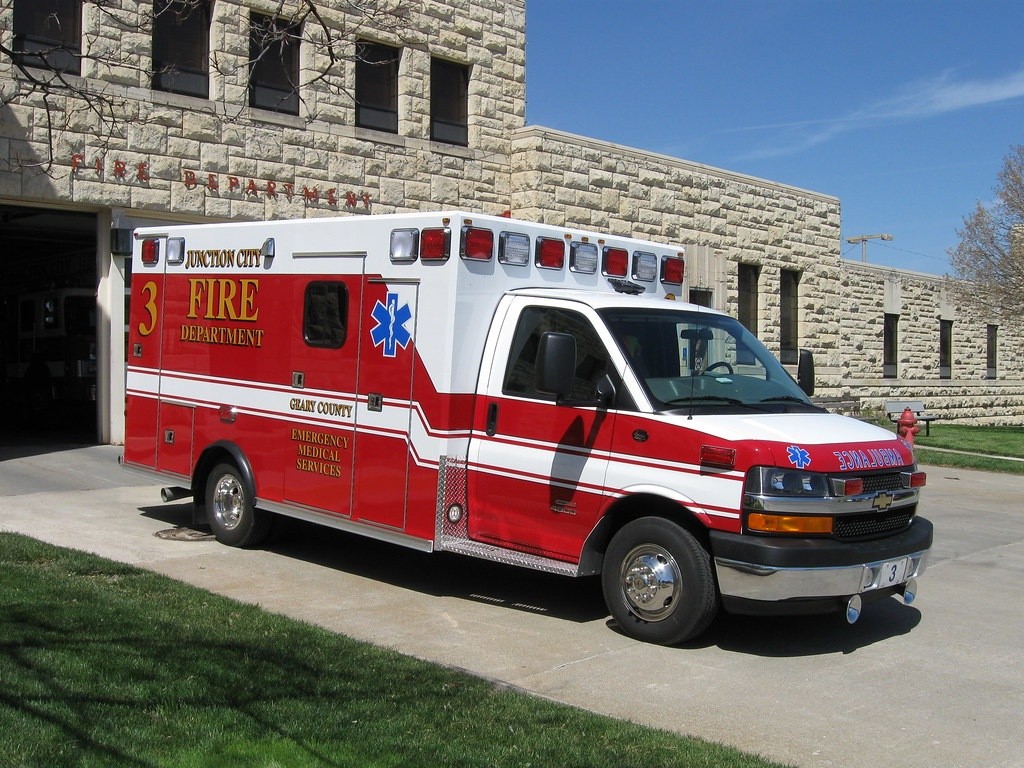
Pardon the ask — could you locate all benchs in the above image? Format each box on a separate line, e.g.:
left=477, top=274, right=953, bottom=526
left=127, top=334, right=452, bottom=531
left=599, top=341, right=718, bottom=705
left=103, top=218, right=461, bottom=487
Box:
left=886, top=400, right=939, bottom=436
left=809, top=396, right=878, bottom=422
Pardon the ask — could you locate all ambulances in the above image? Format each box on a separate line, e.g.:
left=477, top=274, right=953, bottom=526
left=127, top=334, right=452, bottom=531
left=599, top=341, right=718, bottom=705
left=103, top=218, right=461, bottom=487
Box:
left=122, top=212, right=935, bottom=648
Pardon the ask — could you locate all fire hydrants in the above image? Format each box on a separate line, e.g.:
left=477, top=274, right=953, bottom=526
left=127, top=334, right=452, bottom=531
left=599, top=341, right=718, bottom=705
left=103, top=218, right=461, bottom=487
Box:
left=895, top=406, right=922, bottom=456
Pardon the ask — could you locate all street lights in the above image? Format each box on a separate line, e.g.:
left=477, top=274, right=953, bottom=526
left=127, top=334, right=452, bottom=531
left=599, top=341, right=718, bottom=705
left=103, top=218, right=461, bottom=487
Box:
left=843, top=233, right=894, bottom=264
